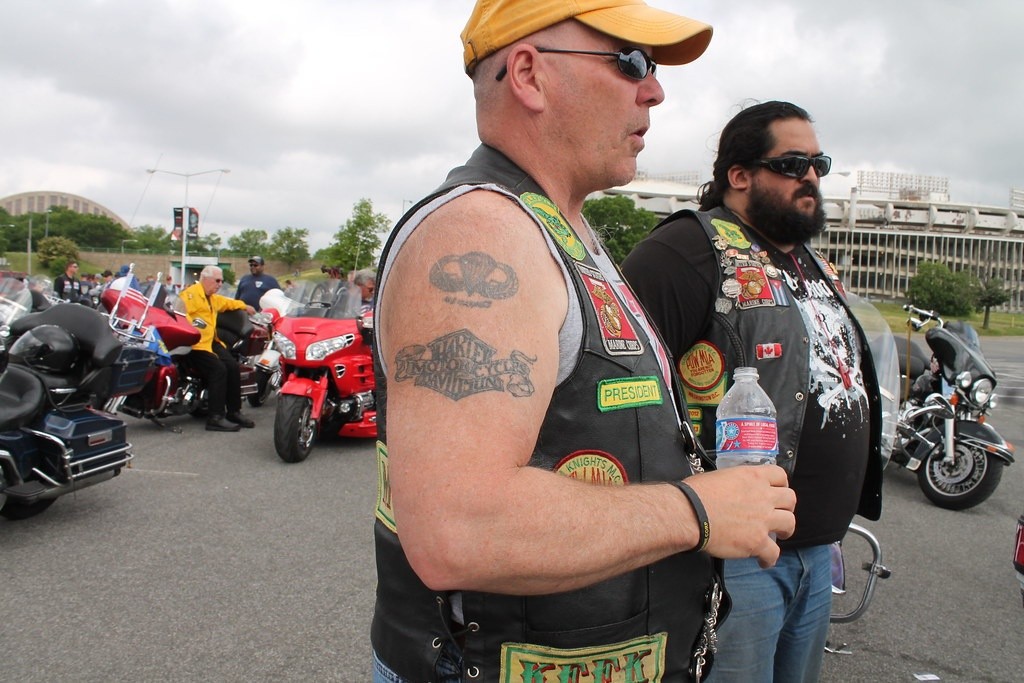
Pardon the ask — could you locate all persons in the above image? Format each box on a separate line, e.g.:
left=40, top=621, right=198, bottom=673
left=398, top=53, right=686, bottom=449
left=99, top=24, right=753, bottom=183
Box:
left=622, top=101, right=882, bottom=683
left=365, top=0, right=798, bottom=683
left=162, top=274, right=180, bottom=296
left=80, top=271, right=102, bottom=307
left=235, top=256, right=281, bottom=313
left=146, top=272, right=155, bottom=282
left=120, top=266, right=142, bottom=291
left=177, top=265, right=255, bottom=431
left=101, top=270, right=120, bottom=291
left=346, top=269, right=375, bottom=329
left=54, top=261, right=82, bottom=303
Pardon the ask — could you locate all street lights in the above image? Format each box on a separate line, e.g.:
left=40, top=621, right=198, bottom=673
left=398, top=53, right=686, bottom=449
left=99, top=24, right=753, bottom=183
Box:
left=146, top=168, right=231, bottom=293
left=402, top=199, right=413, bottom=216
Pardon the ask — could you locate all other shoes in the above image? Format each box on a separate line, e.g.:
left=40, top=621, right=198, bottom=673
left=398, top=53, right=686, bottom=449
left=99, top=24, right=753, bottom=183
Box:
left=226, top=411, right=254, bottom=428
left=206, top=417, right=240, bottom=431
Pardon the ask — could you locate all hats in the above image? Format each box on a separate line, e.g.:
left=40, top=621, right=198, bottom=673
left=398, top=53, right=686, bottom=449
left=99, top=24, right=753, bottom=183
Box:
left=120, top=265, right=129, bottom=276
left=249, top=255, right=265, bottom=265
left=461, top=0, right=714, bottom=81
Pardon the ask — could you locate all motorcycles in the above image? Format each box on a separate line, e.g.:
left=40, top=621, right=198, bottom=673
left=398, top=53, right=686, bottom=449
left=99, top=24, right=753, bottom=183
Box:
left=0, top=301, right=155, bottom=521
left=97, top=262, right=283, bottom=434
left=814, top=288, right=900, bottom=652
left=868, top=302, right=1016, bottom=511
left=260, top=272, right=379, bottom=463
left=0, top=265, right=331, bottom=375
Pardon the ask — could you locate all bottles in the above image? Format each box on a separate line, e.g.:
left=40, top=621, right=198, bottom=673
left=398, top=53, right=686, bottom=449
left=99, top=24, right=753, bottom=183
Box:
left=715, top=367, right=779, bottom=546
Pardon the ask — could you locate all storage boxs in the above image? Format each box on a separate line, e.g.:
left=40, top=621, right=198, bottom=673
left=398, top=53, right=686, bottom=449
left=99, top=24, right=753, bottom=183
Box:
left=42, top=409, right=126, bottom=474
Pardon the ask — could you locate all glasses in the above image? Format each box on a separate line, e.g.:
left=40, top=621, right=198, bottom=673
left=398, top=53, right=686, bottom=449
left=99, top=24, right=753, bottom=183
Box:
left=250, top=264, right=260, bottom=267
left=496, top=47, right=658, bottom=84
left=207, top=277, right=224, bottom=284
left=757, top=154, right=831, bottom=179
left=362, top=285, right=374, bottom=292
left=70, top=266, right=77, bottom=269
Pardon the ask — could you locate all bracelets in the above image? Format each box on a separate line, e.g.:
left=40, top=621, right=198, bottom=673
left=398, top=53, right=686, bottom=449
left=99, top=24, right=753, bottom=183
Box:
left=669, top=479, right=710, bottom=553
